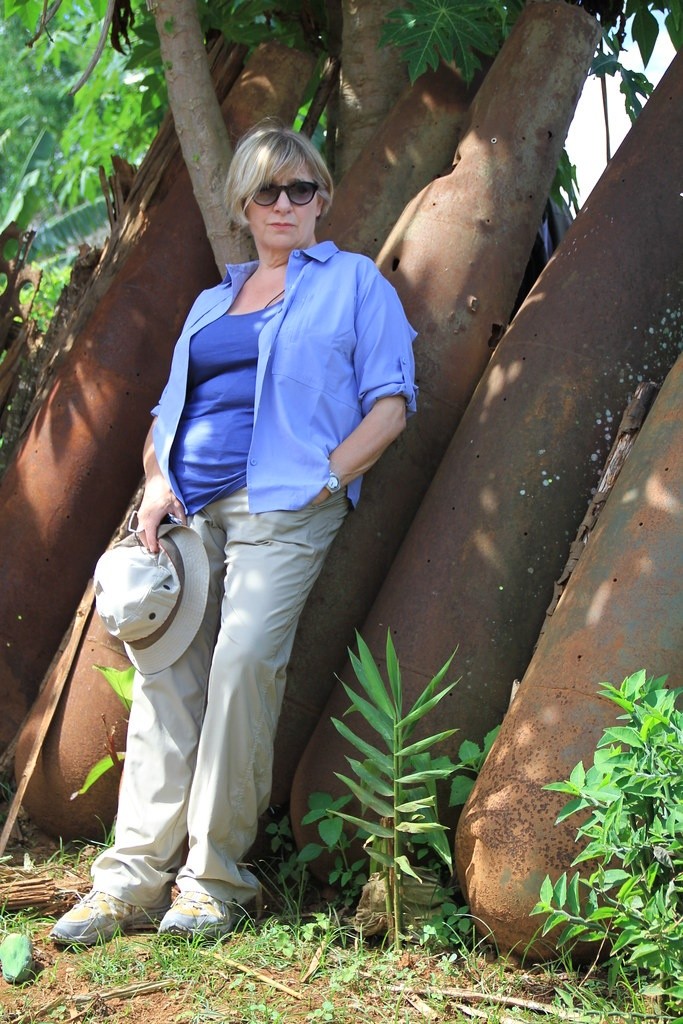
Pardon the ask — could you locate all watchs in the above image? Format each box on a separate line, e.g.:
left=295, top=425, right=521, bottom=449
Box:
left=324, top=470, right=341, bottom=496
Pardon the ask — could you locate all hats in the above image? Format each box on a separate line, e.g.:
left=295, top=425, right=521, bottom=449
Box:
left=93, top=524, right=210, bottom=675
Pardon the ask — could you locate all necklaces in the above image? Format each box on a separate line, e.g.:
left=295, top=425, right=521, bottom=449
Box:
left=264, top=290, right=285, bottom=308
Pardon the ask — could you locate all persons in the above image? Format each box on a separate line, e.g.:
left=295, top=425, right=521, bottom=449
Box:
left=49, top=116, right=418, bottom=946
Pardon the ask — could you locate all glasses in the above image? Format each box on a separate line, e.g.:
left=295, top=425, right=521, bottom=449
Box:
left=252, top=179, right=319, bottom=207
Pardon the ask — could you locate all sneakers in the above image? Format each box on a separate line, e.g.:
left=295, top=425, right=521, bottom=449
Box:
left=48, top=888, right=172, bottom=945
left=156, top=890, right=236, bottom=938
